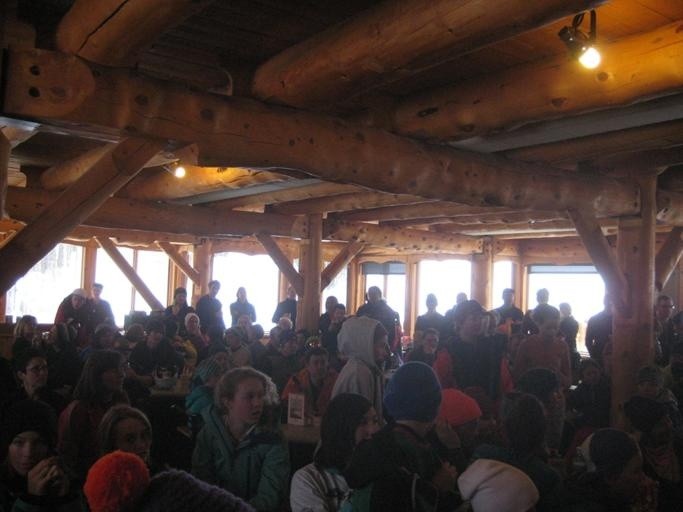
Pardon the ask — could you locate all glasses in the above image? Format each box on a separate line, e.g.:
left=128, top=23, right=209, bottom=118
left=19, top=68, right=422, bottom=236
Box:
left=31, top=365, right=48, bottom=373
left=661, top=305, right=676, bottom=309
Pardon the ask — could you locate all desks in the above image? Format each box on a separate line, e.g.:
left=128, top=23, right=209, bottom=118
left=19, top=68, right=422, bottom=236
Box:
left=146, top=374, right=192, bottom=401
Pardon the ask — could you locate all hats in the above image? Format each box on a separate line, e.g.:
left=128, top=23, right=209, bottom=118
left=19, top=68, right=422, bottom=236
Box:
left=72, top=288, right=87, bottom=299
left=517, top=369, right=561, bottom=408
left=173, top=287, right=187, bottom=299
left=199, top=358, right=222, bottom=384
left=454, top=299, right=491, bottom=324
left=383, top=361, right=442, bottom=423
left=623, top=396, right=669, bottom=432
left=225, top=325, right=245, bottom=339
left=434, top=388, right=483, bottom=427
left=0, top=398, right=57, bottom=463
left=636, top=365, right=665, bottom=390
left=505, top=393, right=547, bottom=445
left=589, top=429, right=639, bottom=481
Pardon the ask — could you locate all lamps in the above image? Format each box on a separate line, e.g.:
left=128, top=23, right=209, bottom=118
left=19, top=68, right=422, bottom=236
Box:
left=161, top=161, right=188, bottom=178
left=558, top=9, right=602, bottom=68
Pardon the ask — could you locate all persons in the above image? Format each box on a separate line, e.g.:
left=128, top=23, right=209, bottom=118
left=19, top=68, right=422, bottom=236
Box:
left=585, top=295, right=612, bottom=357
left=331, top=315, right=390, bottom=433
left=55, top=289, right=87, bottom=324
left=229, top=287, right=256, bottom=328
left=191, top=367, right=290, bottom=511
left=267, top=318, right=309, bottom=389
left=124, top=314, right=203, bottom=397
left=406, top=331, right=438, bottom=366
left=561, top=356, right=683, bottom=512
left=290, top=391, right=378, bottom=511
left=434, top=301, right=508, bottom=411
left=318, top=297, right=339, bottom=333
left=515, top=368, right=561, bottom=419
left=56, top=348, right=129, bottom=453
left=531, top=287, right=561, bottom=321
left=0, top=400, right=78, bottom=511
left=471, top=394, right=559, bottom=497
left=282, top=347, right=337, bottom=419
left=356, top=286, right=394, bottom=315
left=343, top=362, right=460, bottom=511
left=98, top=405, right=152, bottom=467
left=195, top=280, right=225, bottom=333
left=516, top=303, right=570, bottom=385
left=204, top=316, right=266, bottom=367
left=426, top=387, right=482, bottom=461
left=87, top=282, right=115, bottom=322
left=163, top=287, right=189, bottom=316
left=8, top=351, right=61, bottom=408
left=457, top=458, right=539, bottom=511
left=560, top=302, right=578, bottom=354
left=12, top=315, right=35, bottom=350
left=272, top=285, right=298, bottom=331
left=492, top=289, right=522, bottom=332
left=652, top=294, right=683, bottom=355
left=455, top=292, right=468, bottom=306
left=324, top=305, right=347, bottom=345
left=413, top=294, right=443, bottom=335
left=40, top=321, right=124, bottom=363
